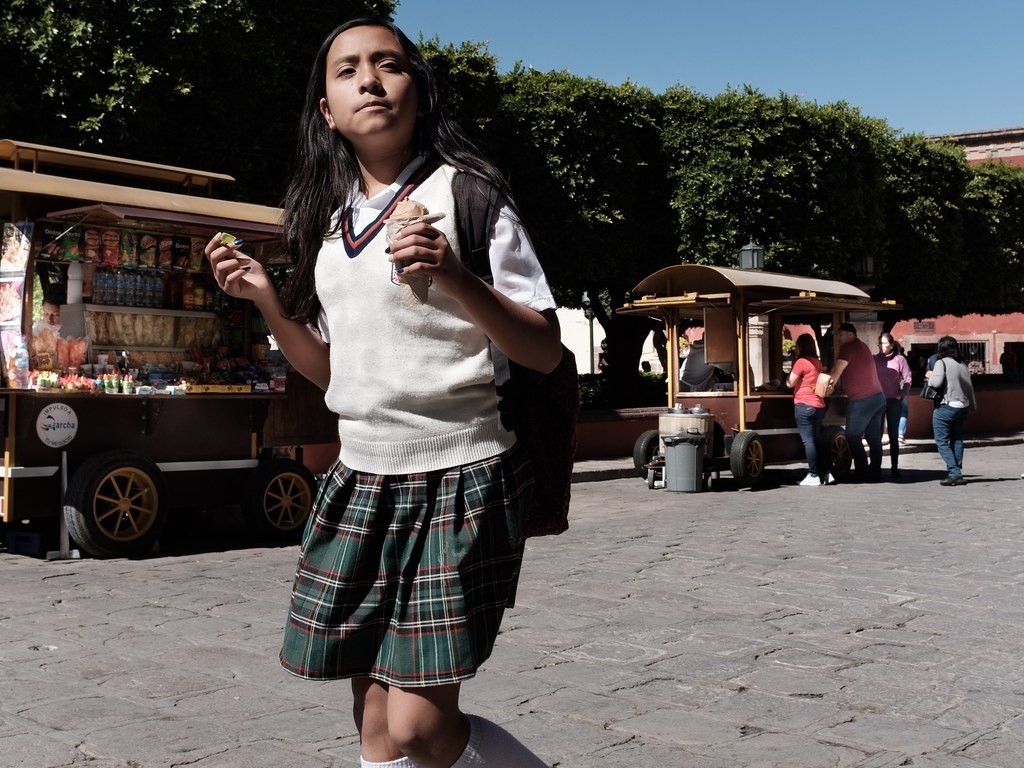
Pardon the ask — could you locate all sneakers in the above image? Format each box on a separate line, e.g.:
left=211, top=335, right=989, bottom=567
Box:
left=796, top=473, right=821, bottom=486
left=823, top=471, right=835, bottom=484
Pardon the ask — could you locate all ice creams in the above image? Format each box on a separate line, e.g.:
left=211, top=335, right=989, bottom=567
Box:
left=389, top=197, right=432, bottom=303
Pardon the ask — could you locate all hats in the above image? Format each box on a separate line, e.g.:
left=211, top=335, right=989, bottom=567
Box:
left=831, top=323, right=856, bottom=335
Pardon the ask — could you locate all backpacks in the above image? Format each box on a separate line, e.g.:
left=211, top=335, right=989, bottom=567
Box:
left=453, top=166, right=579, bottom=536
left=801, top=357, right=833, bottom=398
left=919, top=353, right=946, bottom=400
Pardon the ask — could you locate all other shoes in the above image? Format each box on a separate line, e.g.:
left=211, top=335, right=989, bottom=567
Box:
left=940, top=475, right=966, bottom=486
left=868, top=464, right=882, bottom=472
left=891, top=465, right=898, bottom=473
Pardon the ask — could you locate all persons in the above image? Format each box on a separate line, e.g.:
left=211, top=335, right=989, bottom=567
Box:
left=872, top=332, right=912, bottom=478
left=926, top=353, right=938, bottom=371
left=42, top=292, right=64, bottom=326
left=829, top=323, right=887, bottom=483
left=894, top=340, right=908, bottom=445
left=665, top=332, right=736, bottom=392
left=925, top=336, right=978, bottom=486
left=785, top=333, right=836, bottom=487
left=1000, top=345, right=1020, bottom=385
left=909, top=351, right=926, bottom=388
left=205, top=17, right=567, bottom=768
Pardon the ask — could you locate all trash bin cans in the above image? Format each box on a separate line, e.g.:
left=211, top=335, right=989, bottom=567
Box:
left=660, top=435, right=707, bottom=492
